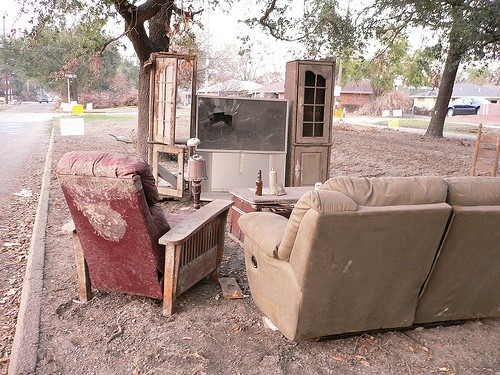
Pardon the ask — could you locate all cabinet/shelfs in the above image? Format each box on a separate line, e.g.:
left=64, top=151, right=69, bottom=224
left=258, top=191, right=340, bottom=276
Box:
left=148, top=53, right=198, bottom=197
left=285, top=59, right=335, bottom=187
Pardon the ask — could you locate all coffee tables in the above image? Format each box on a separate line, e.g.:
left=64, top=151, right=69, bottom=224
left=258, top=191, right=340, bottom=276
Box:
left=228, top=186, right=316, bottom=249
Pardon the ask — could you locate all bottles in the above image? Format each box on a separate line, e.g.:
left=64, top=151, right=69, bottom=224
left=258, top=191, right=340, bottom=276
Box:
left=269, top=171, right=276, bottom=193
left=255, top=170, right=263, bottom=196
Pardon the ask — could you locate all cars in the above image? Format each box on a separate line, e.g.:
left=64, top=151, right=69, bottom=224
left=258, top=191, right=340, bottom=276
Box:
left=39, top=95, right=58, bottom=103
left=446, top=96, right=492, bottom=116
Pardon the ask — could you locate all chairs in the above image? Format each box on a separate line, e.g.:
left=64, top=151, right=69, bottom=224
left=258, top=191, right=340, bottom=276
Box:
left=56, top=151, right=235, bottom=315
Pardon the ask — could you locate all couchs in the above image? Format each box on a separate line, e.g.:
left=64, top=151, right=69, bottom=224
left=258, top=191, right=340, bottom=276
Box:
left=237, top=176, right=500, bottom=341
